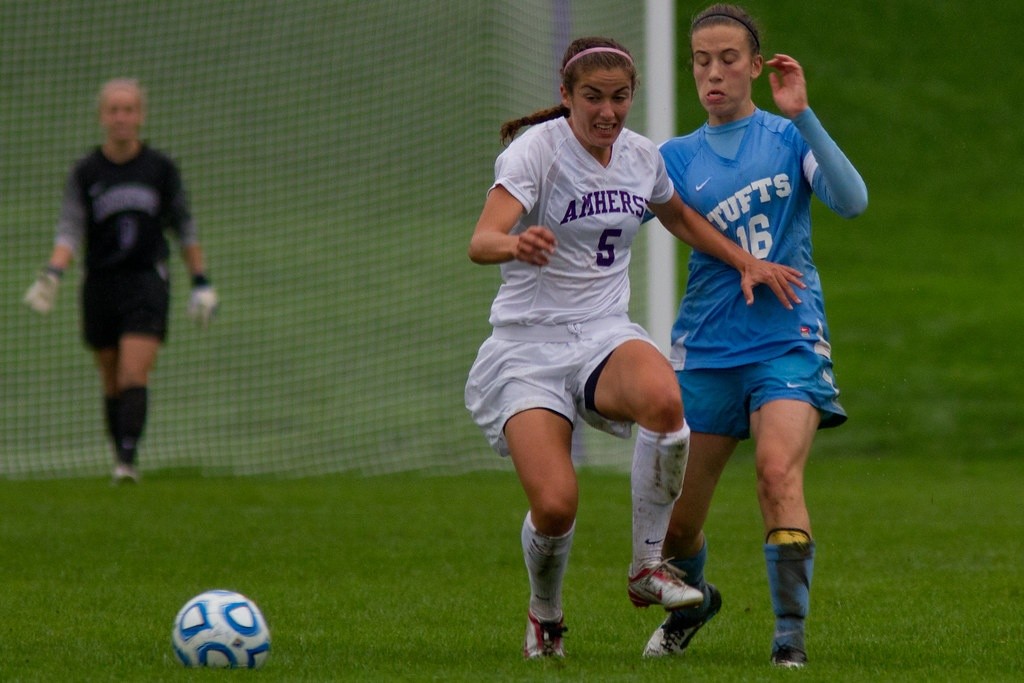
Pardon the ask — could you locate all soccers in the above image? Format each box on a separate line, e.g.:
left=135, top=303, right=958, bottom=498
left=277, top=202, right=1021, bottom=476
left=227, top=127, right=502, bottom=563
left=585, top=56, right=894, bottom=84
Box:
left=172, top=590, right=273, bottom=671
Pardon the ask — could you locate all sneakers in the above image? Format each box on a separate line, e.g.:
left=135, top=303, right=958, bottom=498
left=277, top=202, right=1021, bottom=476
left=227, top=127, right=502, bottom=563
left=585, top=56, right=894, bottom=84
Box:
left=770, top=640, right=809, bottom=670
left=627, top=557, right=703, bottom=611
left=524, top=608, right=568, bottom=661
left=642, top=584, right=722, bottom=658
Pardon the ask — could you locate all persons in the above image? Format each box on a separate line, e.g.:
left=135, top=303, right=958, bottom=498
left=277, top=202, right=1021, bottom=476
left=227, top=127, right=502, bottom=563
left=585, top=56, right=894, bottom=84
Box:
left=28, top=77, right=219, bottom=485
left=641, top=4, right=868, bottom=668
left=463, top=37, right=807, bottom=661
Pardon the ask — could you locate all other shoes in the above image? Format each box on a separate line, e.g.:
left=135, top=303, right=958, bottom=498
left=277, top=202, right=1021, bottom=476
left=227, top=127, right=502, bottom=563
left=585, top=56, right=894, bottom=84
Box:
left=114, top=464, right=136, bottom=483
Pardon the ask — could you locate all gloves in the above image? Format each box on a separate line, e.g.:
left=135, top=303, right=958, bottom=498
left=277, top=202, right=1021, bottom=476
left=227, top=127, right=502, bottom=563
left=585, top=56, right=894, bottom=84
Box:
left=188, top=276, right=218, bottom=329
left=20, top=266, right=60, bottom=318
